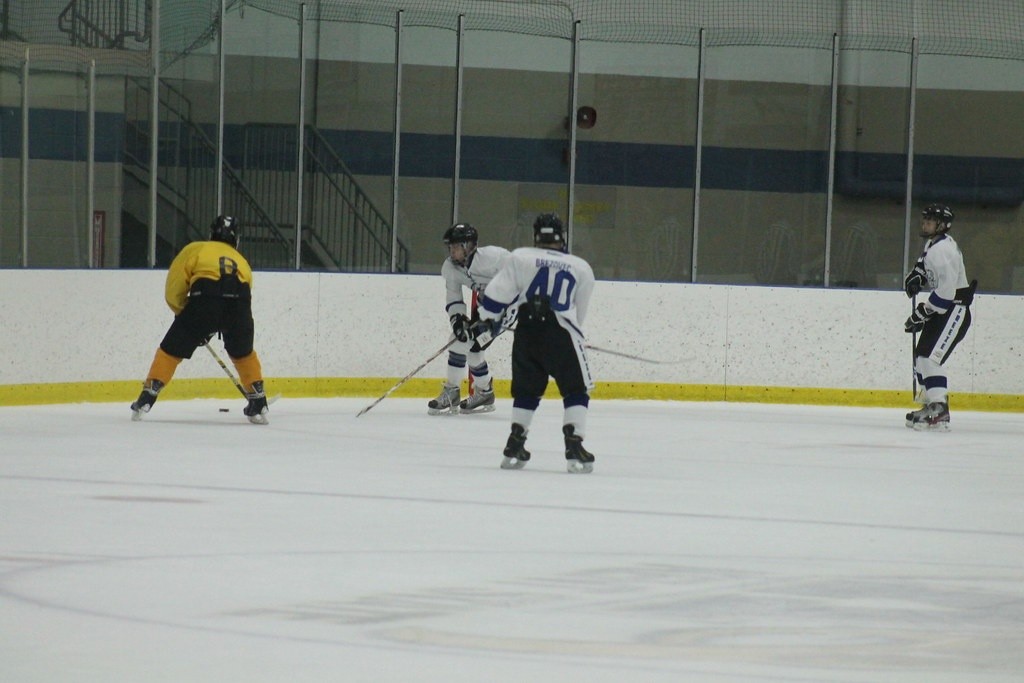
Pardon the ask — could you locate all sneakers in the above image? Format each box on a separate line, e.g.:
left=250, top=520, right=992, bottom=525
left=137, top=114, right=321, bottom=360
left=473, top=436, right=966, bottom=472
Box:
left=459, top=376, right=496, bottom=414
left=244, top=380, right=270, bottom=424
left=131, top=379, right=165, bottom=421
left=906, top=395, right=951, bottom=433
left=562, top=424, right=595, bottom=474
left=500, top=422, right=530, bottom=470
left=428, top=381, right=461, bottom=415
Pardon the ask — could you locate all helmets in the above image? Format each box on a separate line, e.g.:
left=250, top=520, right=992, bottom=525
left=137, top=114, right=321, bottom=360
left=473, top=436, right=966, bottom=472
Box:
left=533, top=212, right=564, bottom=244
left=923, top=204, right=954, bottom=224
left=210, top=215, right=240, bottom=250
left=443, top=222, right=477, bottom=245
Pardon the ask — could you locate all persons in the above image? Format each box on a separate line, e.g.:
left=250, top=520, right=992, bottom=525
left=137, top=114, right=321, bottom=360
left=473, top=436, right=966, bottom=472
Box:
left=427, top=223, right=519, bottom=414
left=904, top=206, right=979, bottom=433
left=479, top=211, right=595, bottom=475
left=132, top=214, right=272, bottom=426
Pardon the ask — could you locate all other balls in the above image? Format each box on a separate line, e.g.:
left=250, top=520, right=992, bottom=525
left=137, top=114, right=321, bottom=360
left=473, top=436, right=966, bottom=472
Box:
left=219, top=408, right=229, bottom=412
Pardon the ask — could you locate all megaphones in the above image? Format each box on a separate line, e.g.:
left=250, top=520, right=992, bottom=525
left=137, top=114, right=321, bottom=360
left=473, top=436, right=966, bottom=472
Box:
left=577, top=106, right=597, bottom=129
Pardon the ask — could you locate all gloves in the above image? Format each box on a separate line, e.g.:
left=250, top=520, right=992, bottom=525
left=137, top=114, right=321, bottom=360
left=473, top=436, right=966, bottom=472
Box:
left=450, top=314, right=473, bottom=343
left=905, top=261, right=927, bottom=298
left=470, top=321, right=496, bottom=353
left=904, top=303, right=937, bottom=332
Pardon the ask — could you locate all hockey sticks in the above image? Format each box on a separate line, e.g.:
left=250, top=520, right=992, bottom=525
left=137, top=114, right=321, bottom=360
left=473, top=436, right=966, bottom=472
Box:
left=355, top=319, right=482, bottom=419
left=204, top=343, right=283, bottom=406
left=910, top=294, right=924, bottom=402
left=475, top=319, right=698, bottom=366
